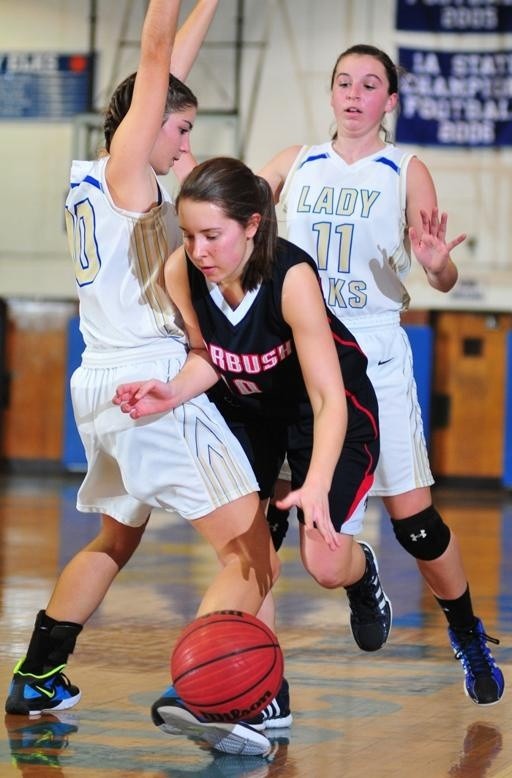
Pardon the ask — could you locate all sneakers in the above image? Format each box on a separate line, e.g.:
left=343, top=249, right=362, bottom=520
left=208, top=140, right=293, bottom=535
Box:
left=268, top=509, right=290, bottom=553
left=446, top=721, right=502, bottom=778
left=5, top=653, right=81, bottom=715
left=447, top=617, right=505, bottom=706
left=346, top=541, right=392, bottom=652
left=150, top=676, right=292, bottom=756
left=4, top=714, right=79, bottom=770
left=159, top=727, right=293, bottom=778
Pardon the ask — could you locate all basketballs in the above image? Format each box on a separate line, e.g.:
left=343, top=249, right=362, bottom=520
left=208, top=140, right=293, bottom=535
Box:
left=171, top=612, right=283, bottom=722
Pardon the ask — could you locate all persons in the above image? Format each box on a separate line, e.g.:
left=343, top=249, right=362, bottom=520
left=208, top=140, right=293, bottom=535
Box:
left=109, top=156, right=393, bottom=732
left=4, top=0, right=283, bottom=757
left=256, top=43, right=505, bottom=707
